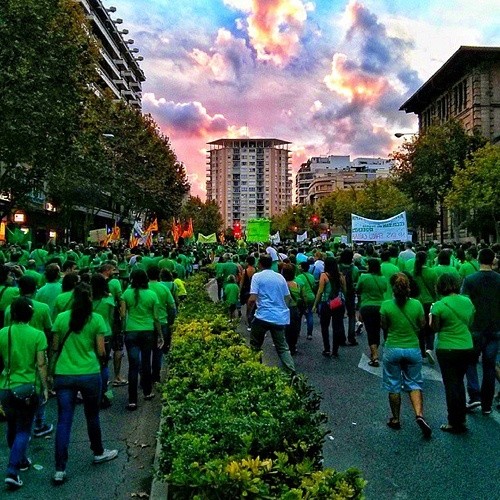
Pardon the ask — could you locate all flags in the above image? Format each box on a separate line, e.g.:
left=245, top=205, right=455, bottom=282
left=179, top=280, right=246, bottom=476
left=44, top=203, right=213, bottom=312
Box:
left=102, top=224, right=120, bottom=246
left=228, top=219, right=242, bottom=237
left=172, top=216, right=193, bottom=243
left=128, top=218, right=158, bottom=248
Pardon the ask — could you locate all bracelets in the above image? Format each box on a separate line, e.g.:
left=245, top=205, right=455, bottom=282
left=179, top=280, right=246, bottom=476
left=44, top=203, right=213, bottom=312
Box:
left=429, top=312, right=432, bottom=317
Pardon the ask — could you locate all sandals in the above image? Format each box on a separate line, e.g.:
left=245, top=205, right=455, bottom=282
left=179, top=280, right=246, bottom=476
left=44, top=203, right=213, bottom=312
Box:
left=369, top=358, right=379, bottom=367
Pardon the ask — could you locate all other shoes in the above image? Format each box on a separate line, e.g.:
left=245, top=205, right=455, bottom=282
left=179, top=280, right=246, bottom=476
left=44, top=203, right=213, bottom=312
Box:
left=348, top=336, right=355, bottom=344
left=467, top=396, right=482, bottom=408
left=340, top=341, right=346, bottom=346
left=425, top=349, right=436, bottom=365
left=482, top=405, right=492, bottom=413
left=113, top=379, right=128, bottom=385
left=145, top=394, right=154, bottom=400
left=307, top=335, right=313, bottom=340
left=355, top=321, right=363, bottom=336
left=417, top=415, right=432, bottom=438
left=332, top=351, right=338, bottom=357
left=126, top=402, right=136, bottom=409
left=461, top=418, right=466, bottom=426
left=387, top=418, right=400, bottom=430
left=422, top=357, right=427, bottom=363
left=442, top=420, right=461, bottom=430
left=76, top=392, right=84, bottom=401
left=322, top=351, right=330, bottom=354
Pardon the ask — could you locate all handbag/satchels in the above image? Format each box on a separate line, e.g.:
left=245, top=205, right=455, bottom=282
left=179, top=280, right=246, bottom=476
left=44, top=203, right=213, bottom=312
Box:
left=240, top=269, right=251, bottom=305
left=298, top=299, right=306, bottom=313
left=8, top=382, right=37, bottom=410
left=327, top=290, right=347, bottom=311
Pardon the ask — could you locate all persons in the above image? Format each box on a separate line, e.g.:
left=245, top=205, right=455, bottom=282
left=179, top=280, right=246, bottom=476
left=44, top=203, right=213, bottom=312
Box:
left=428, top=274, right=476, bottom=433
left=247, top=255, right=296, bottom=373
left=378, top=273, right=431, bottom=435
left=355, top=259, right=387, bottom=363
left=51, top=282, right=118, bottom=481
left=0, top=239, right=500, bottom=436
left=119, top=269, right=164, bottom=411
left=311, top=256, right=346, bottom=357
left=0, top=297, right=48, bottom=486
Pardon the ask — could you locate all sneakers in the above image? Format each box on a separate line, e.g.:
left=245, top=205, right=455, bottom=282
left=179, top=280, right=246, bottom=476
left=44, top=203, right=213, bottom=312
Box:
left=52, top=471, right=66, bottom=481
left=33, top=424, right=53, bottom=436
left=20, top=458, right=31, bottom=471
left=94, top=448, right=118, bottom=463
left=4, top=474, right=23, bottom=486
